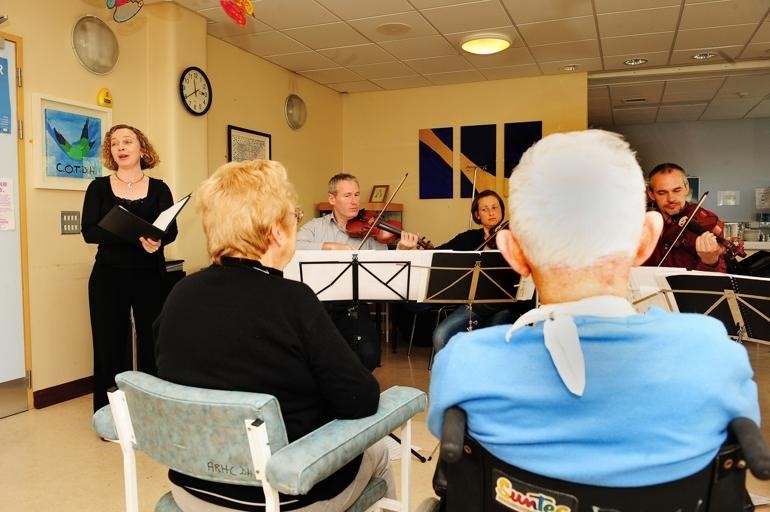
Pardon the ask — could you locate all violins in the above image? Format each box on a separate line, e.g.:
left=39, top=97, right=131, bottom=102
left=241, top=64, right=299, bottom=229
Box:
left=664, top=199, right=748, bottom=259
left=345, top=208, right=435, bottom=251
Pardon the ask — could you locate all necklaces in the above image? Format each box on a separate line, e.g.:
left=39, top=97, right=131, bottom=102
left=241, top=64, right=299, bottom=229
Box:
left=113, top=171, right=148, bottom=193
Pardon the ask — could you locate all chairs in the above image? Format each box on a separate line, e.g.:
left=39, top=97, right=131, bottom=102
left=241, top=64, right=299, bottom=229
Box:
left=107, top=370, right=429, bottom=512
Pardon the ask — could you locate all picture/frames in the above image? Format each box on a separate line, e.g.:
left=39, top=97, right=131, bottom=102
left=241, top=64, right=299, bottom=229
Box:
left=369, top=185, right=390, bottom=203
left=31, top=93, right=113, bottom=192
left=228, top=125, right=271, bottom=163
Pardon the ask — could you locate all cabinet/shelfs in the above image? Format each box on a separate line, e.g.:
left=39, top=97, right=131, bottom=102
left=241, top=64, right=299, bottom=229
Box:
left=318, top=203, right=405, bottom=230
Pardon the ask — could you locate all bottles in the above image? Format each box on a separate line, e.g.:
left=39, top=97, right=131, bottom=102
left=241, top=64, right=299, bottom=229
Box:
left=760, top=232, right=769, bottom=241
left=737, top=231, right=743, bottom=240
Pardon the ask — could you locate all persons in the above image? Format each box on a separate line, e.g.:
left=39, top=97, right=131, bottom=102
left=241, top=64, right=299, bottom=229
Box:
left=291, top=169, right=420, bottom=373
left=644, top=159, right=732, bottom=275
left=423, top=129, right=764, bottom=485
left=431, top=189, right=515, bottom=354
left=82, top=124, right=180, bottom=443
left=149, top=156, right=400, bottom=512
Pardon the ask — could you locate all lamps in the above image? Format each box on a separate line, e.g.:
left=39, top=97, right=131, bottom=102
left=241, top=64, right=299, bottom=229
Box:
left=462, top=32, right=512, bottom=55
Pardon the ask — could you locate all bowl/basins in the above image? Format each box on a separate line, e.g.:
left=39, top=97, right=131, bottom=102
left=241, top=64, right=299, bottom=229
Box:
left=743, top=229, right=760, bottom=240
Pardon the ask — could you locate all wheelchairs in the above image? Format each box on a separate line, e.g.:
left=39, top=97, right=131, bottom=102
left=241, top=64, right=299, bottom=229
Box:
left=414, top=404, right=770, bottom=512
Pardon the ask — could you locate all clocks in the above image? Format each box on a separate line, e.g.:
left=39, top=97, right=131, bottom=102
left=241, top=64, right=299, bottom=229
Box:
left=179, top=65, right=211, bottom=116
